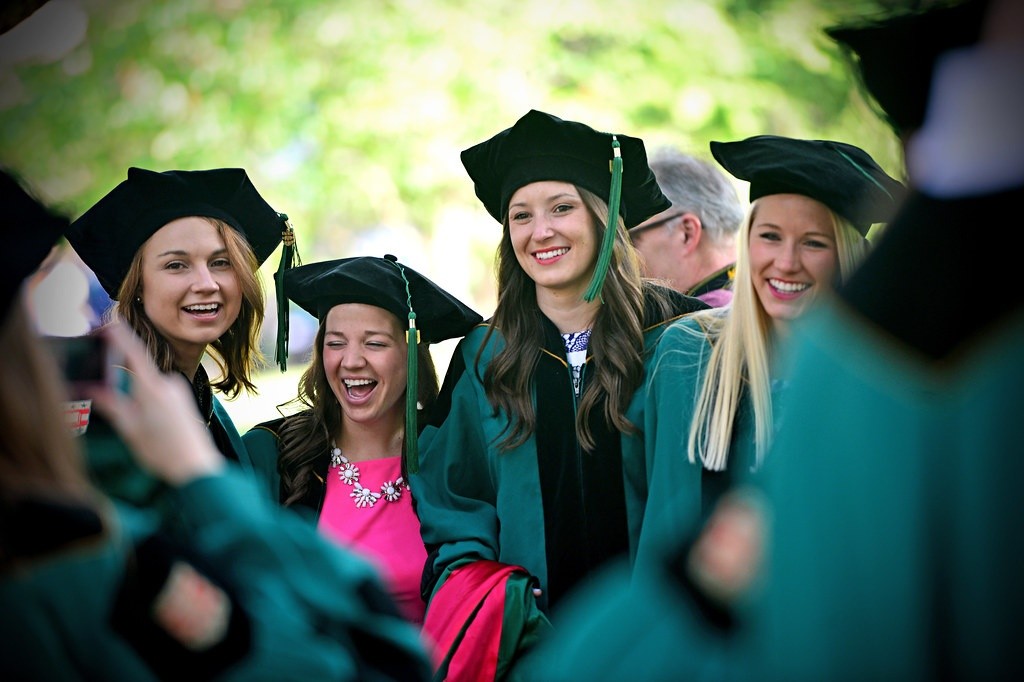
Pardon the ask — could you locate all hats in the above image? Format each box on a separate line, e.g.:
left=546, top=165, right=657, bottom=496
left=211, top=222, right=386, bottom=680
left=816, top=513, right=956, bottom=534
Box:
left=710, top=136, right=909, bottom=238
left=0, top=168, right=70, bottom=322
left=273, top=255, right=484, bottom=473
left=460, top=109, right=673, bottom=303
left=65, top=166, right=296, bottom=373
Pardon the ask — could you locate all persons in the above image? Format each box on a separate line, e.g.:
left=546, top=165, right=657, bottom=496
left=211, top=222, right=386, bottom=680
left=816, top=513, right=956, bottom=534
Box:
left=622, top=143, right=741, bottom=310
left=0, top=168, right=431, bottom=682
left=241, top=256, right=484, bottom=638
left=509, top=0, right=1024, bottom=682
left=397, top=108, right=708, bottom=682
left=67, top=166, right=294, bottom=507
left=623, top=129, right=910, bottom=557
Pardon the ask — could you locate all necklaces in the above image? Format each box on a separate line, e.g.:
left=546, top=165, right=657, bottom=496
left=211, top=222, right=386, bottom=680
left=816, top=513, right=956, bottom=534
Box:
left=323, top=418, right=411, bottom=508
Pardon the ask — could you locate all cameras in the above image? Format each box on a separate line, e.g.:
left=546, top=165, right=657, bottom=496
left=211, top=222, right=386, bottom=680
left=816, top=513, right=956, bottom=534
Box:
left=41, top=335, right=116, bottom=395
